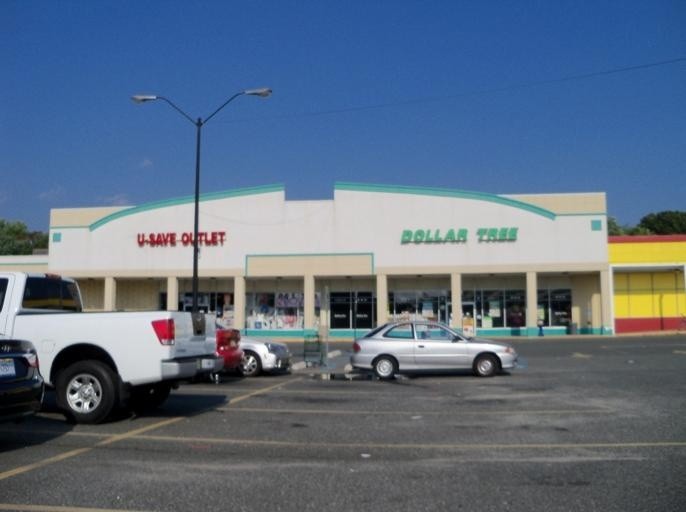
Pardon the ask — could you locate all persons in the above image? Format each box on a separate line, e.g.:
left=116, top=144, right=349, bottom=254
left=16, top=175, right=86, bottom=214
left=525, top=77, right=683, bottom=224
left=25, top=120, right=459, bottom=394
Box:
left=276, top=293, right=320, bottom=306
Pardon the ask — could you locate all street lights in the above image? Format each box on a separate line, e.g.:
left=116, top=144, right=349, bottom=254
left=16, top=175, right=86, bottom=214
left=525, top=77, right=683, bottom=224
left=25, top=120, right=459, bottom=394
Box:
left=131, top=88, right=272, bottom=313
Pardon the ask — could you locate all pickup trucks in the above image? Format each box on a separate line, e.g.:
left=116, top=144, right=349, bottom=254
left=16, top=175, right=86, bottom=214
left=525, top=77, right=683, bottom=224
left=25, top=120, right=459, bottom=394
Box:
left=0, top=273, right=224, bottom=423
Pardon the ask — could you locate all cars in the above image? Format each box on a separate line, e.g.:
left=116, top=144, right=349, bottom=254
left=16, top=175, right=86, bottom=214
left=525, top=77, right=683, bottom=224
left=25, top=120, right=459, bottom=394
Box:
left=351, top=320, right=518, bottom=379
left=188, top=321, right=290, bottom=384
left=0, top=339, right=44, bottom=423
